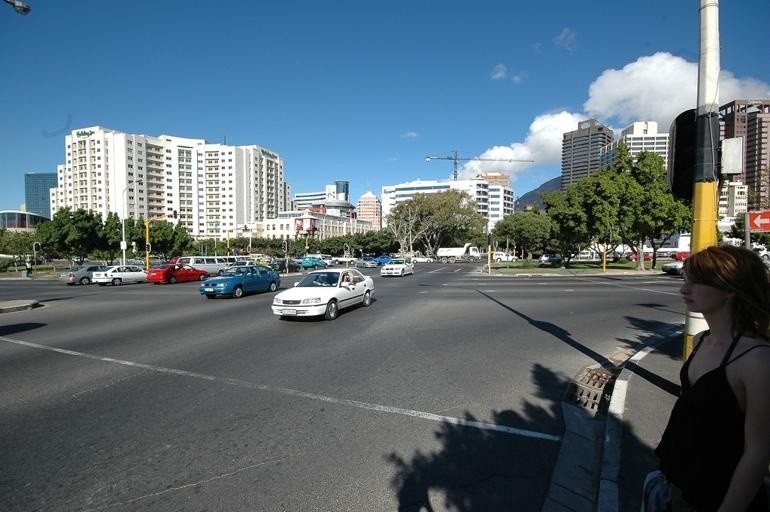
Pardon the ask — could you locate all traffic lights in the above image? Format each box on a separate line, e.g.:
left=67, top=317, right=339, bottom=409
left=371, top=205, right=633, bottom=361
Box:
left=281, top=241, right=287, bottom=253
left=33, top=242, right=41, bottom=254
left=173, top=210, right=177, bottom=219
left=146, top=243, right=151, bottom=253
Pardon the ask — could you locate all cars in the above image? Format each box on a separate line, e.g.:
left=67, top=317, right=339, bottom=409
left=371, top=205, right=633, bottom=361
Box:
left=538, top=253, right=559, bottom=262
left=127, top=247, right=392, bottom=270
left=270, top=268, right=375, bottom=321
left=410, top=254, right=434, bottom=264
left=627, top=246, right=770, bottom=277
left=71, top=255, right=89, bottom=263
left=380, top=258, right=415, bottom=277
left=145, top=262, right=209, bottom=284
left=91, top=264, right=148, bottom=286
left=198, top=265, right=280, bottom=298
left=58, top=264, right=108, bottom=285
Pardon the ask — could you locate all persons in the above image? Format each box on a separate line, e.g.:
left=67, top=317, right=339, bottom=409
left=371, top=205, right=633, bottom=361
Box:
left=645, top=245, right=769, bottom=510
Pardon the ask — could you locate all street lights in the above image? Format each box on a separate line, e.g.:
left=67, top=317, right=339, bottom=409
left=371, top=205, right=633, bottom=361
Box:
left=247, top=202, right=269, bottom=253
left=0, top=0, right=36, bottom=17
left=121, top=179, right=143, bottom=266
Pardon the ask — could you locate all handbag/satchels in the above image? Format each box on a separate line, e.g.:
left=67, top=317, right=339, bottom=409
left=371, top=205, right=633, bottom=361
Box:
left=641, top=470, right=673, bottom=512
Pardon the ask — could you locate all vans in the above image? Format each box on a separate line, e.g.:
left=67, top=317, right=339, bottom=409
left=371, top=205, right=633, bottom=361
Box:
left=493, top=252, right=518, bottom=262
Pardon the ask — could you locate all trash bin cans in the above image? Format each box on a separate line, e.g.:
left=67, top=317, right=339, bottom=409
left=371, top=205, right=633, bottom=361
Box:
left=21, top=269, right=28, bottom=278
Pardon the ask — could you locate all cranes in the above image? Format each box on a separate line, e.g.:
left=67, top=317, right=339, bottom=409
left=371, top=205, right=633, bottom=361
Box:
left=426, top=149, right=535, bottom=182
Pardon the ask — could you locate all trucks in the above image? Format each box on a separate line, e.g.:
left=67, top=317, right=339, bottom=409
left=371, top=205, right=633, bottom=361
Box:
left=436, top=242, right=482, bottom=263
